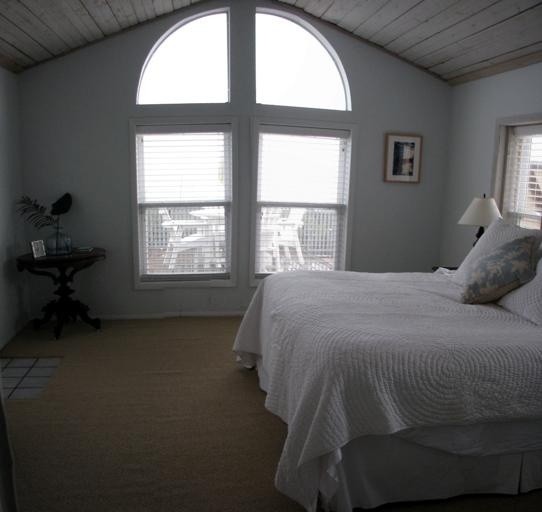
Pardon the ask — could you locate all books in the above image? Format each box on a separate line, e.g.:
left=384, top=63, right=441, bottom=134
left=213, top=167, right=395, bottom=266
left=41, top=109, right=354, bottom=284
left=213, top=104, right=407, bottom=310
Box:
left=77, top=246, right=94, bottom=254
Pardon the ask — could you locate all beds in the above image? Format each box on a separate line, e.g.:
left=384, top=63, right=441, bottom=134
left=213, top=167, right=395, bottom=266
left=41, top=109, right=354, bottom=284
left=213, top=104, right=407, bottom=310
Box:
left=230, top=269, right=542, bottom=512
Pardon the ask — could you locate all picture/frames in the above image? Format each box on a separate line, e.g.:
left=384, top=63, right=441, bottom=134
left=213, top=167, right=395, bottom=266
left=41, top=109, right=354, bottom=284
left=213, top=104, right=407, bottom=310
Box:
left=384, top=131, right=423, bottom=184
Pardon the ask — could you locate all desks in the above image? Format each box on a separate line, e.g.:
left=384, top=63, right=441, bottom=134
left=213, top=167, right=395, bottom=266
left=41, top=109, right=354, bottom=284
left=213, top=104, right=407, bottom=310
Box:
left=17, top=251, right=105, bottom=339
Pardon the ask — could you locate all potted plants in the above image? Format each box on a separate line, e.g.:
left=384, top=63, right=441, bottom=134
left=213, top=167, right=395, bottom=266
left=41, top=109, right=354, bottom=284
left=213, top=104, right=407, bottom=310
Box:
left=17, top=192, right=72, bottom=255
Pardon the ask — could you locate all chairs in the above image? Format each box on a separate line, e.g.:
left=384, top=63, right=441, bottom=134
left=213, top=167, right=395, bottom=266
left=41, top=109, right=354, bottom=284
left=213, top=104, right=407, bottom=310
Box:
left=158, top=207, right=306, bottom=272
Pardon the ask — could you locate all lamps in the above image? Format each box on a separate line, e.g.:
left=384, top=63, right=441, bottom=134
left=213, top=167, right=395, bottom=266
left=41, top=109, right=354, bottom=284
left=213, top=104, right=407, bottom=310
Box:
left=456, top=194, right=502, bottom=247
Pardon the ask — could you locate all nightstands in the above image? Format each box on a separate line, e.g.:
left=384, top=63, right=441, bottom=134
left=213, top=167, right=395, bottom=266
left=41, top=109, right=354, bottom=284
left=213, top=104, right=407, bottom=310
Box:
left=432, top=265, right=459, bottom=272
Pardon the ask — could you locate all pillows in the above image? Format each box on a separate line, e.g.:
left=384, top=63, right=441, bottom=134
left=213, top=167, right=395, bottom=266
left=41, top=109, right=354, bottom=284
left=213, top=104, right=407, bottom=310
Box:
left=455, top=219, right=542, bottom=328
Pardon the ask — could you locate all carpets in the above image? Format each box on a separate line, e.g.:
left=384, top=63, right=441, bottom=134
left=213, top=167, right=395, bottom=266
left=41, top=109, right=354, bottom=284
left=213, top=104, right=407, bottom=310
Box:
left=1, top=315, right=305, bottom=512
